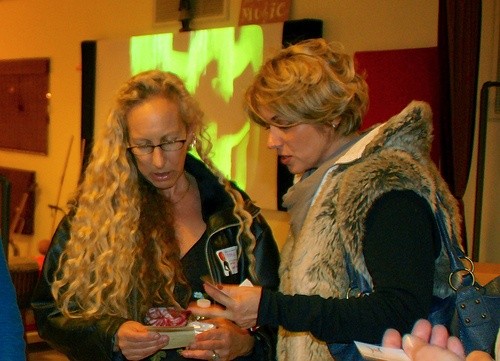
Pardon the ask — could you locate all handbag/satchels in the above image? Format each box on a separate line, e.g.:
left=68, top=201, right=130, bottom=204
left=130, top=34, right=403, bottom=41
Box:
left=327, top=198, right=499, bottom=361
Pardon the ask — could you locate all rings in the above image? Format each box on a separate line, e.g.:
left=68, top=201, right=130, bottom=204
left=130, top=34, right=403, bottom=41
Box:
left=212, top=350, right=220, bottom=361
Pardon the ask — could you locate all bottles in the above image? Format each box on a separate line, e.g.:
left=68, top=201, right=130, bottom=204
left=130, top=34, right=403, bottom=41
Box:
left=187, top=298, right=217, bottom=337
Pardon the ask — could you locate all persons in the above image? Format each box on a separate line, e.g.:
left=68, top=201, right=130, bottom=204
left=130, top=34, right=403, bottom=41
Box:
left=372, top=316, right=467, bottom=361
left=32, top=70, right=280, bottom=361
left=186, top=39, right=464, bottom=361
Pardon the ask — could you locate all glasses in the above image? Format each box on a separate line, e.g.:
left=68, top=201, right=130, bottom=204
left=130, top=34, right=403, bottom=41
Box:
left=125, top=124, right=187, bottom=156
